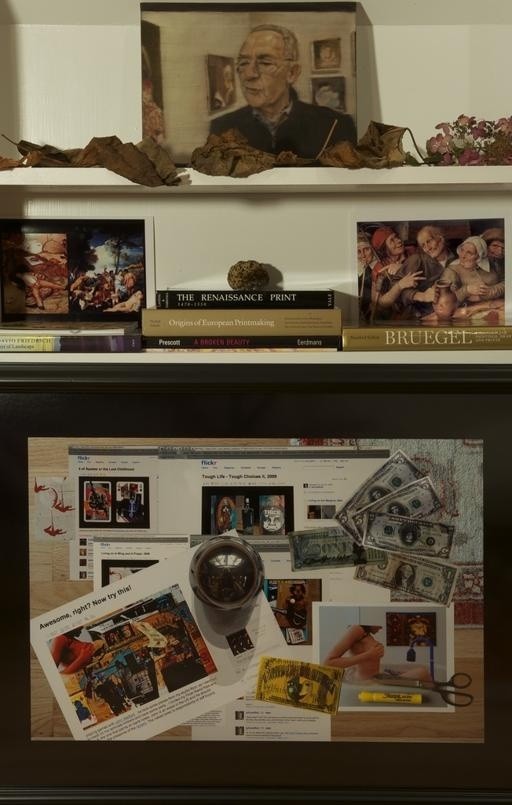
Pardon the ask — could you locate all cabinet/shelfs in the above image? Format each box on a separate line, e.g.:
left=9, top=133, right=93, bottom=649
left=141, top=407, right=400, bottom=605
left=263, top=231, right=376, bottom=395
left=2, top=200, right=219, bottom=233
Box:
left=0, top=2, right=507, bottom=360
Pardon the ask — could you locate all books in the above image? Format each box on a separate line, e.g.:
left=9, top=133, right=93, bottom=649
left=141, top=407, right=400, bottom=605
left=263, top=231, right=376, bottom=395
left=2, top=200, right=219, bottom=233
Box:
left=0, top=287, right=512, bottom=353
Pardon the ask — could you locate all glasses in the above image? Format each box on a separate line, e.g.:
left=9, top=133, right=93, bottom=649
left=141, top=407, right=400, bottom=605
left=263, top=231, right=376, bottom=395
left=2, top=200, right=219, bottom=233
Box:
left=237, top=57, right=297, bottom=74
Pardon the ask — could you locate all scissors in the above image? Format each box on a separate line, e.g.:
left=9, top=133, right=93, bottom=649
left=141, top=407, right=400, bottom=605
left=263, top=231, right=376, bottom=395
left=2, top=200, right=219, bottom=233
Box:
left=356, top=691, right=431, bottom=703
left=377, top=672, right=474, bottom=711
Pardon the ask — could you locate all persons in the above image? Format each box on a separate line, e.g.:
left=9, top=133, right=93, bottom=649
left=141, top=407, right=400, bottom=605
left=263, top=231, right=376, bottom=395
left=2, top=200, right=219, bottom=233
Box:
left=209, top=24, right=358, bottom=167
left=50, top=483, right=432, bottom=683
left=357, top=225, right=505, bottom=322
left=15, top=266, right=144, bottom=312
